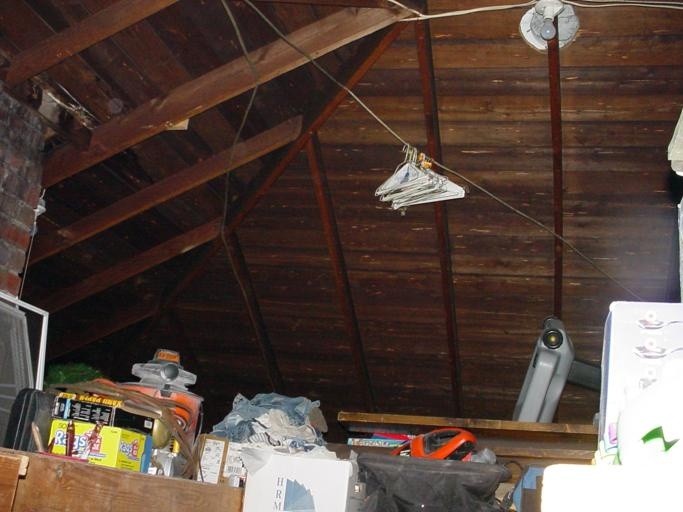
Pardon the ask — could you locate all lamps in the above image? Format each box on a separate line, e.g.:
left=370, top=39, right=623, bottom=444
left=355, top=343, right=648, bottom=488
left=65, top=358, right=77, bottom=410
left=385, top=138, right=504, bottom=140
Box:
left=535, top=0, right=564, bottom=40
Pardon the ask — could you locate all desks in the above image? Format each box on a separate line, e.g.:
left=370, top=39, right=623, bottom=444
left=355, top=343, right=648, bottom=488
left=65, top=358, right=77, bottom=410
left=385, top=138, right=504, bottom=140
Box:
left=337, top=412, right=598, bottom=467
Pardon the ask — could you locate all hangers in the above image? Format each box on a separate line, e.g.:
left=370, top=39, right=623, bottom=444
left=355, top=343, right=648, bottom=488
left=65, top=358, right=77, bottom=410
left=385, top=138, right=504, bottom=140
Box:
left=374, top=143, right=465, bottom=210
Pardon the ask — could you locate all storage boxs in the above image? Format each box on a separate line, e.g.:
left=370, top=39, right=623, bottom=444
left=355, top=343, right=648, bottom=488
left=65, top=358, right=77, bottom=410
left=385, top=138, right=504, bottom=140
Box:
left=194, top=434, right=228, bottom=485
left=48, top=392, right=159, bottom=474
